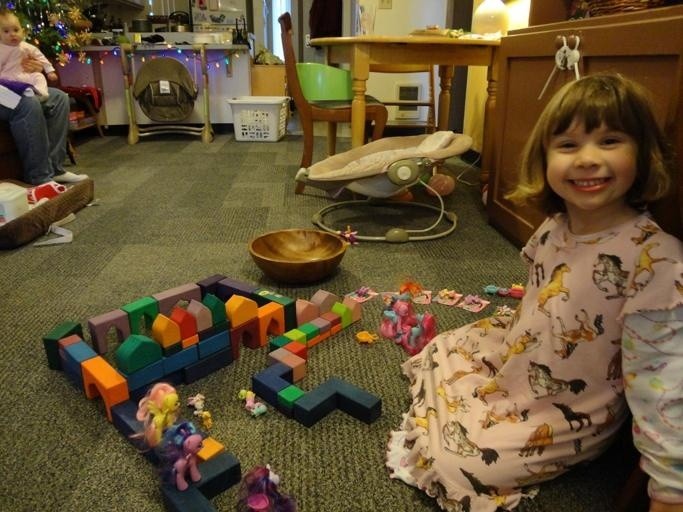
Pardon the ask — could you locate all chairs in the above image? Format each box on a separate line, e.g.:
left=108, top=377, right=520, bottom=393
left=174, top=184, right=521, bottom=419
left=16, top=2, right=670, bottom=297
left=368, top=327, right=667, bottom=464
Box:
left=43, top=71, right=105, bottom=166
left=278, top=12, right=388, bottom=194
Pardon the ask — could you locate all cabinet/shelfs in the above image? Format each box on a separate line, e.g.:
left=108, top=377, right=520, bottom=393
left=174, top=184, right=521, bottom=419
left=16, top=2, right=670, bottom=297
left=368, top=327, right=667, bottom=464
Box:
left=251, top=65, right=291, bottom=119
left=486, top=0, right=683, bottom=252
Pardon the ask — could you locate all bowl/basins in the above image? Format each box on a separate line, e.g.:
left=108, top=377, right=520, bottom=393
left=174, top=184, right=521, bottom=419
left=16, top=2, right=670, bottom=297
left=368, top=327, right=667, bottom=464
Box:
left=250, top=228, right=346, bottom=284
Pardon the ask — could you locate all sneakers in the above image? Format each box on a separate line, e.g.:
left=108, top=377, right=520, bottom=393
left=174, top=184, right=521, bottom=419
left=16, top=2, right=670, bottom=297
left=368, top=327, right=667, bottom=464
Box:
left=52, top=171, right=89, bottom=182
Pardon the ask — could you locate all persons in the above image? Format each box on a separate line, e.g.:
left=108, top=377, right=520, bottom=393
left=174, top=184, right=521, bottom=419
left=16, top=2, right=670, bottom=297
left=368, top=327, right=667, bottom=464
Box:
left=0, top=59, right=92, bottom=191
left=401, top=67, right=682, bottom=512
left=0, top=10, right=54, bottom=107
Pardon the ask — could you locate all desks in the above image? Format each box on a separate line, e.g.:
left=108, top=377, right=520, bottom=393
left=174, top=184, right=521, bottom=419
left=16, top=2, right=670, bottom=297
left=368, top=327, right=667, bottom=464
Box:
left=310, top=36, right=501, bottom=200
